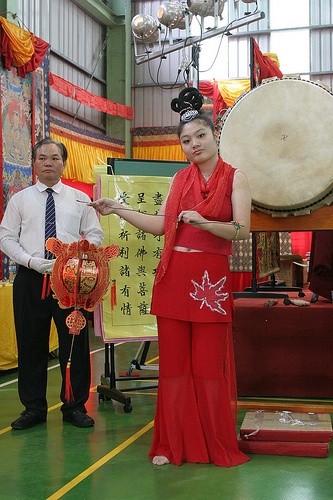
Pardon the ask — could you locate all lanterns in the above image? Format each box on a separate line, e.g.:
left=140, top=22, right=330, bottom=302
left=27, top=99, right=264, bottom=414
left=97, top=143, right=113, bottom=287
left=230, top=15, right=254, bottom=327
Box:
left=44, top=238, right=119, bottom=337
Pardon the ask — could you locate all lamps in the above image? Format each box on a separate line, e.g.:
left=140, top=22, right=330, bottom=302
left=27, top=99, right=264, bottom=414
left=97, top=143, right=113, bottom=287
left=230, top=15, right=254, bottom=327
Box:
left=130, top=13, right=162, bottom=57
left=186, top=0, right=225, bottom=34
left=157, top=1, right=193, bottom=46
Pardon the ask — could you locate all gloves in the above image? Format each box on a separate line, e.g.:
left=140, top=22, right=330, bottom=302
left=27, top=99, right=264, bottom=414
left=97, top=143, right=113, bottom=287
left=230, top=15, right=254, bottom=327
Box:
left=29, top=257, right=53, bottom=274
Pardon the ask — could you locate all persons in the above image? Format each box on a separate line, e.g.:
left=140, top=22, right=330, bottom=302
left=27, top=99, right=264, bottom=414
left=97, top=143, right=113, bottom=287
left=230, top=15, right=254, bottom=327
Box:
left=0, top=137, right=102, bottom=429
left=87, top=87, right=251, bottom=466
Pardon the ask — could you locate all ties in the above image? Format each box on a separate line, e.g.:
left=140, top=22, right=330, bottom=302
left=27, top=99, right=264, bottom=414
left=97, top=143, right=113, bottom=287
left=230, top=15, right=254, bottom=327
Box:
left=43, top=187, right=57, bottom=260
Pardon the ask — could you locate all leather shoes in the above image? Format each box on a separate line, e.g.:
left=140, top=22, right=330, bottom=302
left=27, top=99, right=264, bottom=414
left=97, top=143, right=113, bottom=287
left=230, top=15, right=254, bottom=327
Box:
left=10, top=410, right=48, bottom=430
left=62, top=409, right=96, bottom=428
